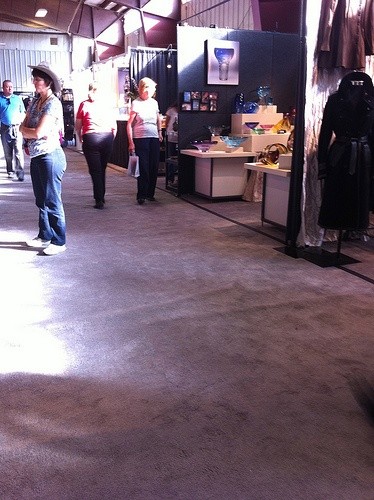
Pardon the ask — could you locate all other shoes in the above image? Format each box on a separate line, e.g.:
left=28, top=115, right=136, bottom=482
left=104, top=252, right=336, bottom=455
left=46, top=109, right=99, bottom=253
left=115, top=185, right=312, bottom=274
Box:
left=7, top=172, right=14, bottom=178
left=137, top=197, right=145, bottom=203
left=145, top=196, right=155, bottom=201
left=18, top=174, right=25, bottom=180
left=95, top=200, right=104, bottom=209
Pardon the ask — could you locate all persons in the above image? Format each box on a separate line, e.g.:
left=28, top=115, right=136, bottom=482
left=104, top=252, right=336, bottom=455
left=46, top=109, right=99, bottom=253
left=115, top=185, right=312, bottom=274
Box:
left=18, top=61, right=66, bottom=255
left=165, top=101, right=178, bottom=183
left=76, top=81, right=117, bottom=209
left=127, top=78, right=163, bottom=203
left=0, top=80, right=27, bottom=180
left=271, top=106, right=296, bottom=134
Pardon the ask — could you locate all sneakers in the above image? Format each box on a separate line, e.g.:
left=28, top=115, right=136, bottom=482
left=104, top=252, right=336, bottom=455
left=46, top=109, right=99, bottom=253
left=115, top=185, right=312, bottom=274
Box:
left=25, top=236, right=51, bottom=247
left=42, top=243, right=67, bottom=254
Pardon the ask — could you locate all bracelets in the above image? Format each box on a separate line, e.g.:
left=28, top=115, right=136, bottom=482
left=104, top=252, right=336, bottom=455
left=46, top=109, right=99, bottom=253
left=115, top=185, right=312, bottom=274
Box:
left=159, top=130, right=162, bottom=131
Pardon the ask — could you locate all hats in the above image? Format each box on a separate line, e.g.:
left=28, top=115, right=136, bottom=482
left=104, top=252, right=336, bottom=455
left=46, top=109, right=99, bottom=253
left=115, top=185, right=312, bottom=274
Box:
left=27, top=61, right=62, bottom=92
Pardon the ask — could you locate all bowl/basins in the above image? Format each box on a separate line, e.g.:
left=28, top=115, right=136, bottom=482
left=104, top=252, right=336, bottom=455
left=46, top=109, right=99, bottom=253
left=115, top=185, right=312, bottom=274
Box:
left=209, top=127, right=223, bottom=136
left=189, top=140, right=218, bottom=153
left=245, top=122, right=260, bottom=129
left=219, top=136, right=248, bottom=147
left=260, top=125, right=275, bottom=131
left=252, top=129, right=265, bottom=135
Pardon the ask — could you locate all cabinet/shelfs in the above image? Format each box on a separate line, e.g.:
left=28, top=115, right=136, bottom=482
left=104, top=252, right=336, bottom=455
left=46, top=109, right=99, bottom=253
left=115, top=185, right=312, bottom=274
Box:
left=165, top=106, right=292, bottom=229
